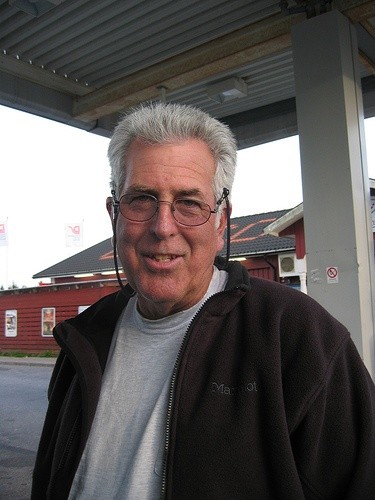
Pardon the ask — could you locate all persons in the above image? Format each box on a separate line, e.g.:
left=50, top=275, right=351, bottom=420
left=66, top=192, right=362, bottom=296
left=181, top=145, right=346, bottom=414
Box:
left=29, top=102, right=375, bottom=499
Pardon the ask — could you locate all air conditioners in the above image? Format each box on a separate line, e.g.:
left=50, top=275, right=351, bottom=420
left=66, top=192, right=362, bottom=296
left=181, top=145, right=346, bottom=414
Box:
left=278, top=253, right=307, bottom=278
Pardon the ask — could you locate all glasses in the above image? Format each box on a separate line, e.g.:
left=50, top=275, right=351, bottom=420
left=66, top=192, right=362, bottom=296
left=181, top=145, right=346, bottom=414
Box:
left=111, top=189, right=230, bottom=227
